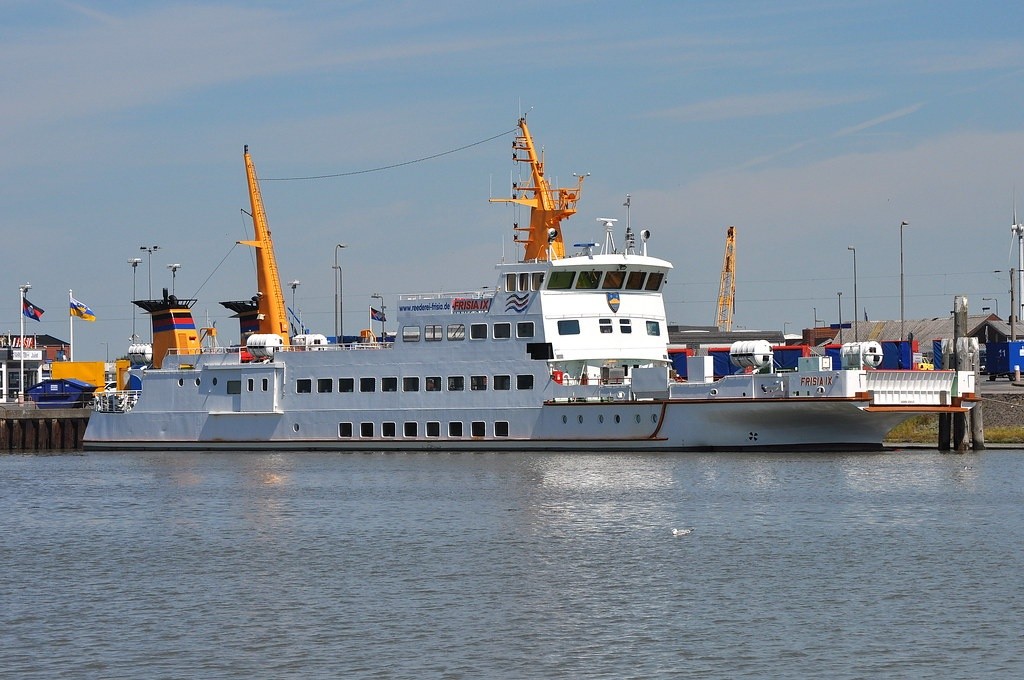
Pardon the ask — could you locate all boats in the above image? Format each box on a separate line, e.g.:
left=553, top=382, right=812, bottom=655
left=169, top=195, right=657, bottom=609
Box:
left=83, top=94, right=981, bottom=452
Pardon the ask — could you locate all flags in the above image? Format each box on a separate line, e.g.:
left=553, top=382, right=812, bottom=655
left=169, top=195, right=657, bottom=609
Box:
left=69, top=298, right=96, bottom=322
left=372, top=308, right=388, bottom=321
left=22, top=297, right=45, bottom=322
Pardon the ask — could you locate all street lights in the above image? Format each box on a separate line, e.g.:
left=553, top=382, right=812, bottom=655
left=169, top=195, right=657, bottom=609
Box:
left=900, top=221, right=908, bottom=339
left=848, top=246, right=858, bottom=340
left=140, top=244, right=162, bottom=344
left=126, top=257, right=142, bottom=343
left=370, top=294, right=386, bottom=345
left=166, top=263, right=182, bottom=296
left=286, top=279, right=302, bottom=336
left=332, top=241, right=346, bottom=345
left=836, top=292, right=845, bottom=344
